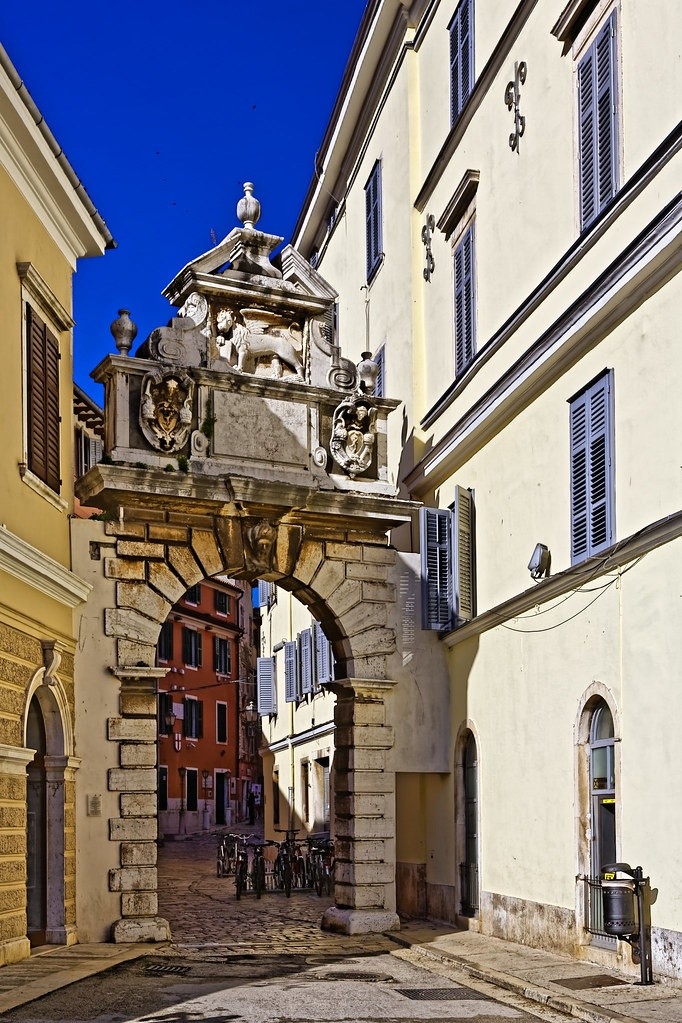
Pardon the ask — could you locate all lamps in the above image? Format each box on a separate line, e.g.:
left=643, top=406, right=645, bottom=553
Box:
left=273, top=638, right=287, bottom=652
left=528, top=543, right=549, bottom=580
left=205, top=625, right=214, bottom=630
left=161, top=709, right=176, bottom=739
left=245, top=701, right=263, bottom=738
left=174, top=615, right=182, bottom=620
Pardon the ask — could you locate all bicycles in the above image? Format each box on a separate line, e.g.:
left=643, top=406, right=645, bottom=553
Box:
left=210, top=827, right=338, bottom=900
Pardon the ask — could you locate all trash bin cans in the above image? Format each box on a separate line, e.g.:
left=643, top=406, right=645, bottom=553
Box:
left=600, top=863, right=655, bottom=986
